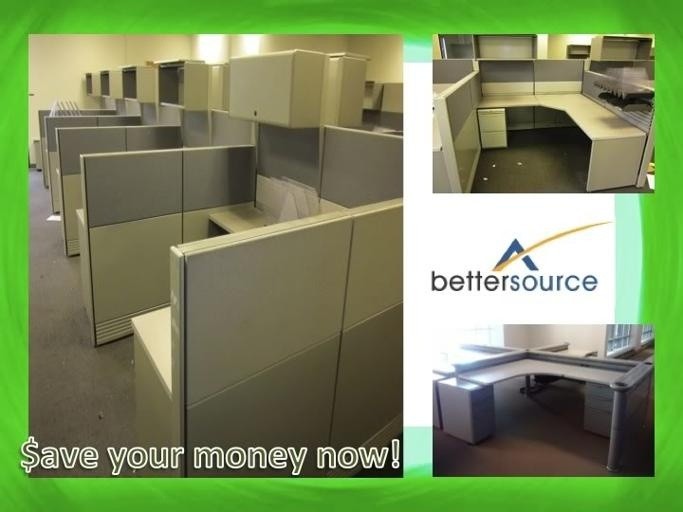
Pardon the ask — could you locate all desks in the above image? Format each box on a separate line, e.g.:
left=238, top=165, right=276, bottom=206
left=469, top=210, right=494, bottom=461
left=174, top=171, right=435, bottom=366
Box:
left=433, top=324, right=655, bottom=473
left=433, top=34, right=655, bottom=192
